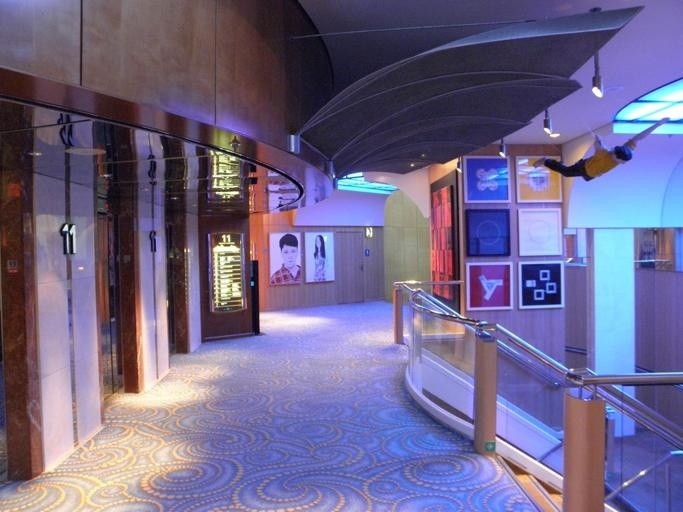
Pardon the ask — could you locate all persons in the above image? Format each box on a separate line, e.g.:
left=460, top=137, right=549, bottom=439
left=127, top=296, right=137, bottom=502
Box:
left=269, top=233, right=301, bottom=286
left=532, top=114, right=672, bottom=182
left=309, top=234, right=328, bottom=282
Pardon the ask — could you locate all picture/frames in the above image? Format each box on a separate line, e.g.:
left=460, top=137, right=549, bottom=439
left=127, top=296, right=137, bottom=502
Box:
left=462, top=155, right=564, bottom=312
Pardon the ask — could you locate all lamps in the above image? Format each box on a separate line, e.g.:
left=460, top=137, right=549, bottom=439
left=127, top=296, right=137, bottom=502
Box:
left=591, top=52, right=602, bottom=99
left=498, top=138, right=505, bottom=159
left=542, top=109, right=552, bottom=135
